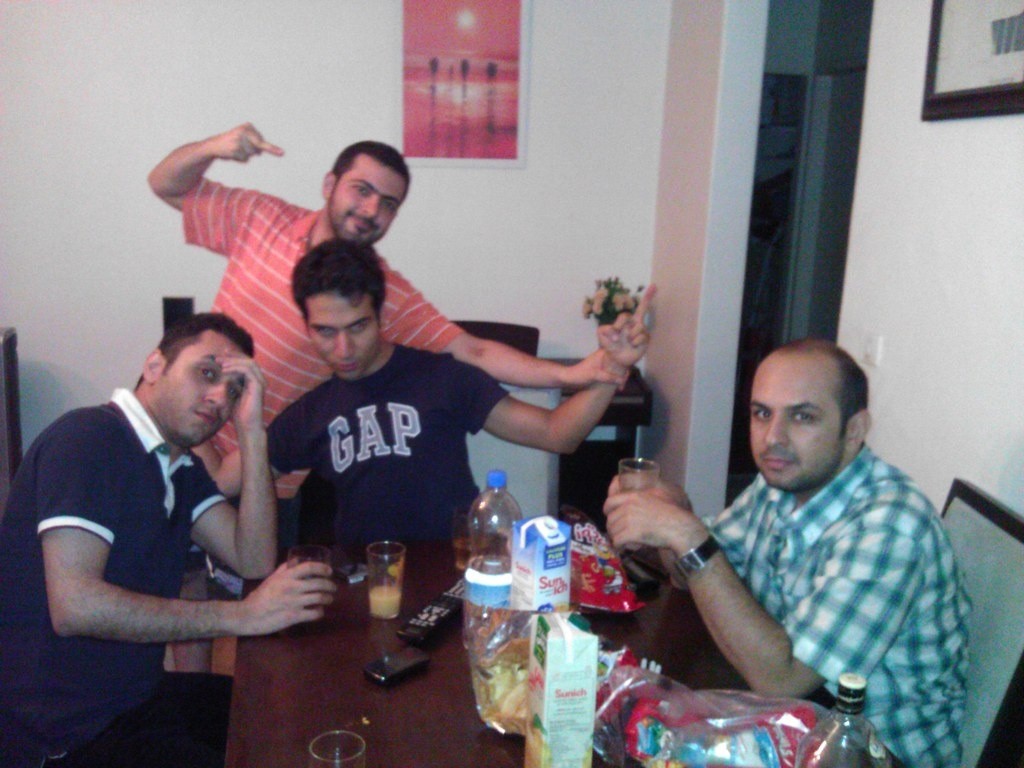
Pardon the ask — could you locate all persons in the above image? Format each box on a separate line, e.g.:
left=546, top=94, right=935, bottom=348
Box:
left=149, top=122, right=628, bottom=672
left=0, top=312, right=337, bottom=768
left=603, top=338, right=975, bottom=768
left=194, top=237, right=656, bottom=549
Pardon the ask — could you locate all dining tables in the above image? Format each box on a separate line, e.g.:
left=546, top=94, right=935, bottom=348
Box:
left=222, top=540, right=906, bottom=768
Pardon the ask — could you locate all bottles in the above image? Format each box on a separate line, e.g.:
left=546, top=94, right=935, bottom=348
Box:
left=466, top=471, right=522, bottom=651
left=794, top=674, right=892, bottom=768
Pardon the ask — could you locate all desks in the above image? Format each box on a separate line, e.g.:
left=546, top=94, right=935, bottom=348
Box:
left=546, top=359, right=652, bottom=528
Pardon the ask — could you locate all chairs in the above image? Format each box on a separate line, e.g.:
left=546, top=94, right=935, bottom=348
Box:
left=940, top=479, right=1024, bottom=768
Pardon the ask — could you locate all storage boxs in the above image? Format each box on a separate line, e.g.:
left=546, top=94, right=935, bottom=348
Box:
left=512, top=514, right=572, bottom=612
left=523, top=611, right=600, bottom=768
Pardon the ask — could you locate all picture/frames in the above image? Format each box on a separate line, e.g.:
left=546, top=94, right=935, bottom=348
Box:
left=922, top=0, right=1024, bottom=121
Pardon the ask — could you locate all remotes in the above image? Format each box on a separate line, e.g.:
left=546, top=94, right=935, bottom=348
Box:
left=396, top=578, right=464, bottom=644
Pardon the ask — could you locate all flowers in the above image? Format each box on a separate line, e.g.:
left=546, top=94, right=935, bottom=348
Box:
left=583, top=271, right=647, bottom=324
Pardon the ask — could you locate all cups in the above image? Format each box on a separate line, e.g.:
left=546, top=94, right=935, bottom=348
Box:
left=368, top=541, right=406, bottom=618
left=308, top=730, right=366, bottom=768
left=619, top=457, right=660, bottom=491
left=286, top=544, right=332, bottom=567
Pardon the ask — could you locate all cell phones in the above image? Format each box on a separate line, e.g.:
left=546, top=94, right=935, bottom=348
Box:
left=362, top=645, right=430, bottom=685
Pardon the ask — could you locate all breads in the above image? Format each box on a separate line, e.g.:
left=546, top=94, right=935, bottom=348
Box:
left=472, top=638, right=531, bottom=735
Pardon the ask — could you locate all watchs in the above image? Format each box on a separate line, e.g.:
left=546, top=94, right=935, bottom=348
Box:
left=676, top=536, right=719, bottom=576
